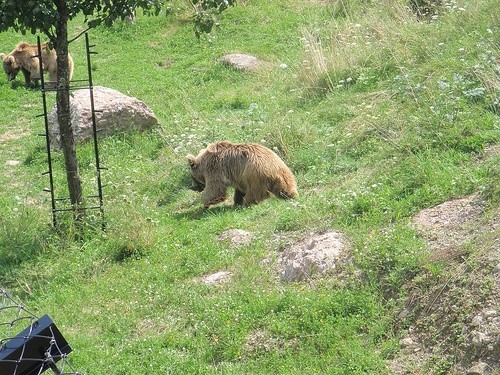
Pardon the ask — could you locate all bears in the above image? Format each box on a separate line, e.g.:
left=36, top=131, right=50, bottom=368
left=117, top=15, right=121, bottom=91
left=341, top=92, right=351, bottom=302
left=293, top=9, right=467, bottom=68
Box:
left=186, top=140, right=299, bottom=208
left=0, top=41, right=74, bottom=87
